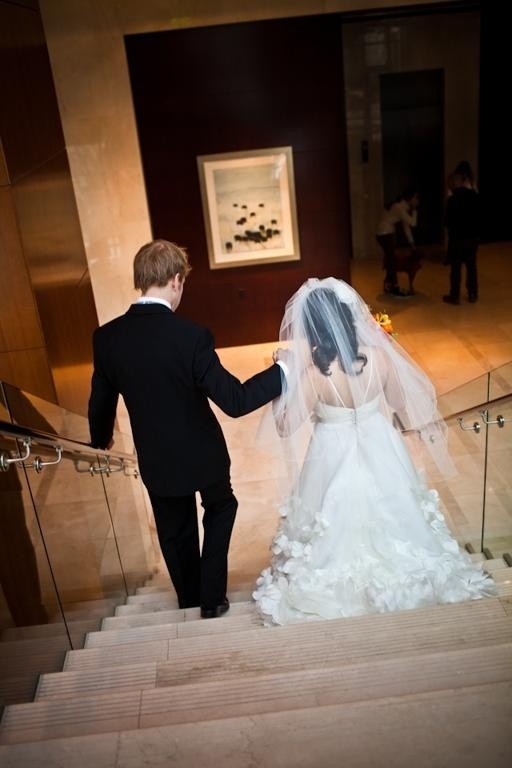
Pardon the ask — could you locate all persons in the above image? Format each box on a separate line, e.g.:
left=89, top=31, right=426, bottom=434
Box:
left=441, top=161, right=481, bottom=264
left=441, top=167, right=480, bottom=306
left=250, top=275, right=496, bottom=626
left=85, top=237, right=302, bottom=620
left=0, top=376, right=62, bottom=627
left=374, top=187, right=419, bottom=296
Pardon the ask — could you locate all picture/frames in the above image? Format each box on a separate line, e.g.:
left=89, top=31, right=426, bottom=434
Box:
left=196, top=146, right=302, bottom=270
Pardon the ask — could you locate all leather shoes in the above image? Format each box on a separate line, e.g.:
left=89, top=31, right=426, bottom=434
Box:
left=202, top=596, right=229, bottom=616
left=442, top=295, right=477, bottom=305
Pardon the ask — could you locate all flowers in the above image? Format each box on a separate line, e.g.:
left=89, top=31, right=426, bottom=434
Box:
left=367, top=305, right=398, bottom=343
